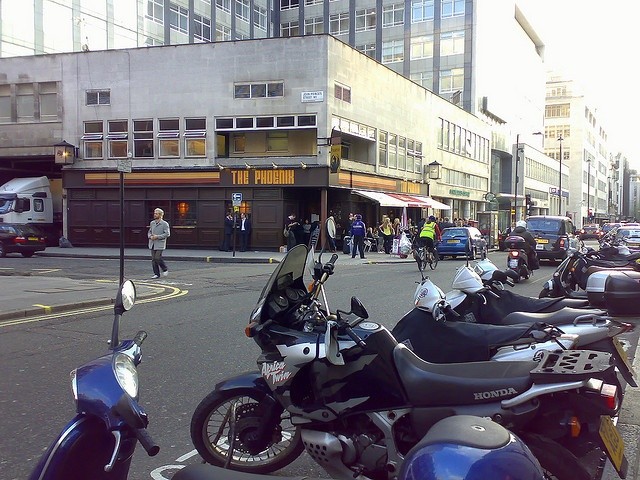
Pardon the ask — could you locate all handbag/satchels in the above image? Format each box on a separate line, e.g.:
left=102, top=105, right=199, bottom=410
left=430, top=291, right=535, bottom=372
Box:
left=284, top=221, right=292, bottom=237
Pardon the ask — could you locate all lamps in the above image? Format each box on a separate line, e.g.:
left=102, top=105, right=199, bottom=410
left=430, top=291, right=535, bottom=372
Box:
left=53, top=139, right=79, bottom=166
left=244, top=162, right=253, bottom=172
left=271, top=160, right=279, bottom=170
left=429, top=159, right=442, bottom=180
left=300, top=160, right=307, bottom=169
left=216, top=163, right=225, bottom=172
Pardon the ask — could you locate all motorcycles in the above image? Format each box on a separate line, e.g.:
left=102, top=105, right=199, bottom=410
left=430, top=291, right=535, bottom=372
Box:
left=599, top=242, right=640, bottom=261
left=473, top=247, right=640, bottom=317
left=538, top=239, right=640, bottom=300
left=570, top=242, right=640, bottom=291
left=191, top=244, right=629, bottom=479
left=445, top=239, right=610, bottom=325
left=505, top=235, right=540, bottom=283
left=28, top=279, right=545, bottom=480
left=390, top=250, right=637, bottom=409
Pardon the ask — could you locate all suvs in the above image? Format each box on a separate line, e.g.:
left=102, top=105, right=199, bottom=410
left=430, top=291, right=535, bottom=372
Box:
left=600, top=222, right=624, bottom=241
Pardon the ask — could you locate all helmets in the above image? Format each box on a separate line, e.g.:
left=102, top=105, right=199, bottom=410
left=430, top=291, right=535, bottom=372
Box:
left=515, top=220, right=527, bottom=231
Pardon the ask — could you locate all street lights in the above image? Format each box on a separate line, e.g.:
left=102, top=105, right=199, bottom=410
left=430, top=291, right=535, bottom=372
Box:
left=585, top=157, right=592, bottom=222
left=515, top=131, right=542, bottom=227
left=607, top=175, right=611, bottom=222
left=557, top=134, right=564, bottom=216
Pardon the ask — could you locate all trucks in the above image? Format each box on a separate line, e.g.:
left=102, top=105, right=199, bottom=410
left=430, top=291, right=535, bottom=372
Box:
left=0, top=176, right=63, bottom=242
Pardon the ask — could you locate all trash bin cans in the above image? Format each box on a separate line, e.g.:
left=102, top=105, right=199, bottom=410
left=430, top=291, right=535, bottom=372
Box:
left=343, top=238, right=351, bottom=254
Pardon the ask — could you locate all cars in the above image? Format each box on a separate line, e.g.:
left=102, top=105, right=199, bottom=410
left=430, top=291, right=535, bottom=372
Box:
left=580, top=224, right=602, bottom=241
left=616, top=226, right=640, bottom=244
left=0, top=223, right=48, bottom=257
left=436, top=226, right=488, bottom=259
left=525, top=215, right=580, bottom=261
left=498, top=227, right=511, bottom=248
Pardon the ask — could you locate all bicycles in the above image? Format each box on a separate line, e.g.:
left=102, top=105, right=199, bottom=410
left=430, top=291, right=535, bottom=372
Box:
left=416, top=240, right=444, bottom=272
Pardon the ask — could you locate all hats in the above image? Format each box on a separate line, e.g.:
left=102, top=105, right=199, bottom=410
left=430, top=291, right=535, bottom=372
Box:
left=357, top=214, right=362, bottom=218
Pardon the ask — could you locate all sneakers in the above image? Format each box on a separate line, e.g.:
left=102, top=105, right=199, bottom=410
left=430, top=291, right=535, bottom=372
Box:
left=163, top=271, right=168, bottom=277
left=150, top=274, right=158, bottom=279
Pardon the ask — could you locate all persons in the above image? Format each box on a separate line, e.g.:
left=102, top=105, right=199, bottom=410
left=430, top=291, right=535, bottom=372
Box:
left=284, top=211, right=300, bottom=251
left=443, top=218, right=451, bottom=229
left=148, top=208, right=170, bottom=279
left=327, top=209, right=337, bottom=252
left=367, top=226, right=376, bottom=252
left=416, top=219, right=427, bottom=236
left=349, top=214, right=359, bottom=256
left=377, top=221, right=384, bottom=253
left=238, top=212, right=251, bottom=251
left=219, top=211, right=233, bottom=252
left=457, top=218, right=462, bottom=227
left=337, top=211, right=346, bottom=249
left=351, top=215, right=366, bottom=258
left=420, top=215, right=441, bottom=260
left=463, top=218, right=469, bottom=227
left=452, top=218, right=457, bottom=227
left=436, top=217, right=439, bottom=224
left=303, top=219, right=311, bottom=246
left=509, top=220, right=537, bottom=269
left=438, top=218, right=444, bottom=232
left=346, top=213, right=353, bottom=236
left=379, top=218, right=394, bottom=254
left=393, top=218, right=401, bottom=239
